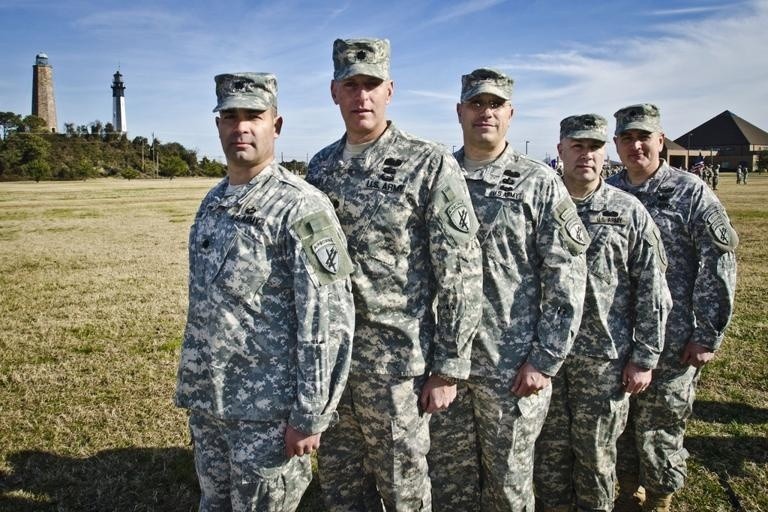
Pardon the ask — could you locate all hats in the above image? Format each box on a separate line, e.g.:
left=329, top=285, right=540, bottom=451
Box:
left=210, top=71, right=279, bottom=113
left=613, top=102, right=665, bottom=140
left=461, top=68, right=515, bottom=102
left=560, top=113, right=610, bottom=144
left=332, top=38, right=390, bottom=82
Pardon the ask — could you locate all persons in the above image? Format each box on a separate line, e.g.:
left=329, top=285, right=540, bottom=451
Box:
left=541, top=113, right=673, bottom=511
left=175, top=72, right=355, bottom=511
left=694, top=159, right=754, bottom=189
left=428, top=69, right=586, bottom=512
left=305, top=40, right=485, bottom=512
left=608, top=104, right=739, bottom=512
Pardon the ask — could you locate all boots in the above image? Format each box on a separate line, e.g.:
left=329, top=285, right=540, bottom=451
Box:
left=612, top=487, right=645, bottom=510
left=641, top=491, right=672, bottom=508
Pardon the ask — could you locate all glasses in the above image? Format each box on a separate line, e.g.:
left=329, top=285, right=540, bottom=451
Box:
left=461, top=98, right=510, bottom=113
